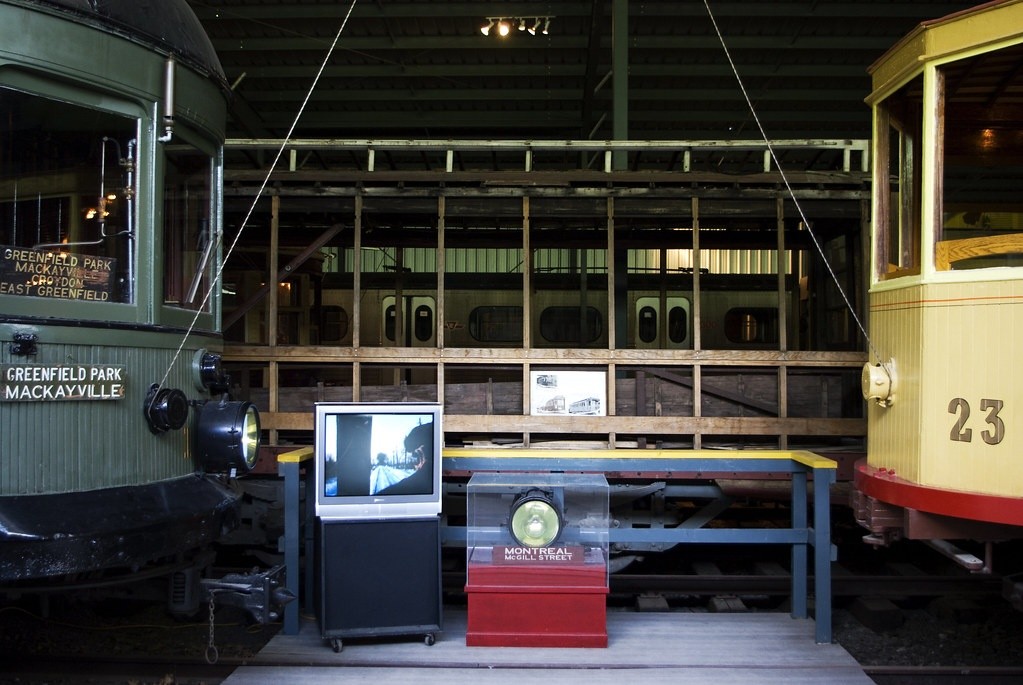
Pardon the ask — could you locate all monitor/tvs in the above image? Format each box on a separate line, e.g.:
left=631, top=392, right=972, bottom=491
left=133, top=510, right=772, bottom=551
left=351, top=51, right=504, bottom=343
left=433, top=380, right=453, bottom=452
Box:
left=314, top=401, right=443, bottom=520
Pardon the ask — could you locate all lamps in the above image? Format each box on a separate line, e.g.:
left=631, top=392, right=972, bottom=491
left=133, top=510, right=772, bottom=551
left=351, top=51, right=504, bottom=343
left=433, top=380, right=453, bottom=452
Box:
left=481, top=16, right=556, bottom=37
